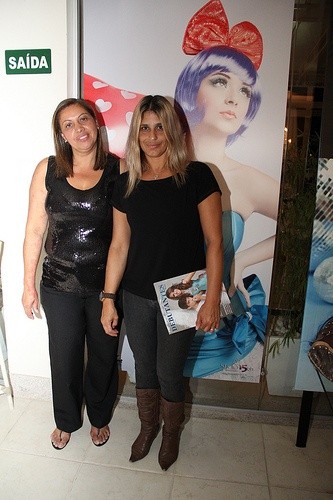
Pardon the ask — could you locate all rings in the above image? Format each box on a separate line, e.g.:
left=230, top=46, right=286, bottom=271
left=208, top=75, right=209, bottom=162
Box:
left=210, top=329, right=214, bottom=331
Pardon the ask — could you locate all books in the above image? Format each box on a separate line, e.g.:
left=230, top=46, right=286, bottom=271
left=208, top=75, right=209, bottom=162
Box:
left=154, top=269, right=232, bottom=335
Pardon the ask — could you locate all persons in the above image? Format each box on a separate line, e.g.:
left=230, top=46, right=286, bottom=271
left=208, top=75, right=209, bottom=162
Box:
left=102, top=95, right=223, bottom=470
left=174, top=0, right=280, bottom=378
left=178, top=292, right=230, bottom=312
left=166, top=271, right=207, bottom=300
left=22, top=98, right=129, bottom=450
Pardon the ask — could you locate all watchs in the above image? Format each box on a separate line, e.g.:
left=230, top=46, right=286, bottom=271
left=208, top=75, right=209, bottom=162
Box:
left=99, top=290, right=116, bottom=301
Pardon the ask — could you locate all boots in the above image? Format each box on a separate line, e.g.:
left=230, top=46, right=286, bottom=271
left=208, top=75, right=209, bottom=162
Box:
left=157, top=398, right=185, bottom=471
left=129, top=386, right=162, bottom=463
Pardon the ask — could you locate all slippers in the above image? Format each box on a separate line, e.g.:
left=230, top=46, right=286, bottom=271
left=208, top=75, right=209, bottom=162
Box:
left=51, top=428, right=71, bottom=449
left=91, top=425, right=112, bottom=447
